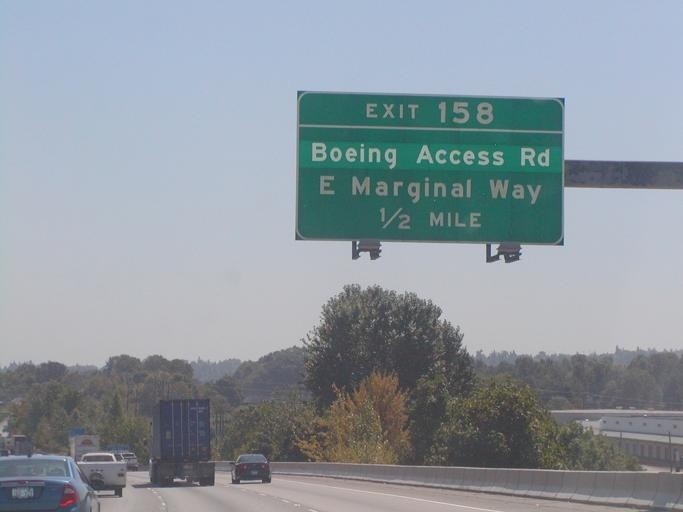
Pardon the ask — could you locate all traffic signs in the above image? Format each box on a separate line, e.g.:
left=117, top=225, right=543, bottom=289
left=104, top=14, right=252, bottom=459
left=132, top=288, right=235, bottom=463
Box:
left=297, top=90, right=563, bottom=244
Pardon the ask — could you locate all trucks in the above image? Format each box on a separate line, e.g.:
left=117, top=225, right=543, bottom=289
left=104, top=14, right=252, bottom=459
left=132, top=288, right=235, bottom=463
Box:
left=149, top=399, right=215, bottom=486
left=70, top=435, right=100, bottom=462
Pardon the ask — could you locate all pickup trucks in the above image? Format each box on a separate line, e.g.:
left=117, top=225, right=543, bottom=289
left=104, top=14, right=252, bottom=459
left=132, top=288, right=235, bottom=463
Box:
left=78, top=453, right=127, bottom=496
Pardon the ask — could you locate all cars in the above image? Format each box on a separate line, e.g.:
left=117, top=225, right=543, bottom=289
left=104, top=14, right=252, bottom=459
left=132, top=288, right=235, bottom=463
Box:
left=0, top=454, right=100, bottom=512
left=229, top=454, right=271, bottom=484
left=122, top=452, right=138, bottom=470
